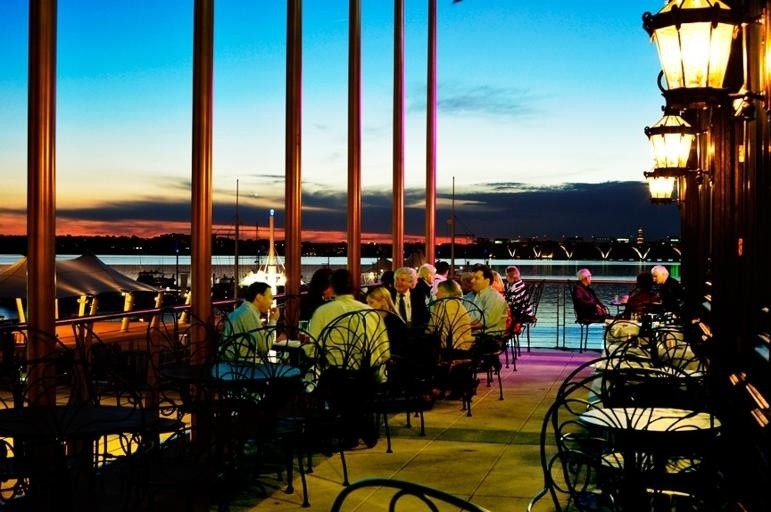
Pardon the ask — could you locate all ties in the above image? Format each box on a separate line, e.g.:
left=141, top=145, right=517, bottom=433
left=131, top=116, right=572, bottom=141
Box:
left=398, top=292, right=408, bottom=324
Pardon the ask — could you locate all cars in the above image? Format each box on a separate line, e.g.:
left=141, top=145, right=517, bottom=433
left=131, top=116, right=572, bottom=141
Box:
left=136, top=270, right=175, bottom=292
left=210, top=276, right=236, bottom=300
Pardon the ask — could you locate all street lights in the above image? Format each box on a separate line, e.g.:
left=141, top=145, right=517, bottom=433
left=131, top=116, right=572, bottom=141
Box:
left=135, top=245, right=144, bottom=279
left=174, top=247, right=183, bottom=298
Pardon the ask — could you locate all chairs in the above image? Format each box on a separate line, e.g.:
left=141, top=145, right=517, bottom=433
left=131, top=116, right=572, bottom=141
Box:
left=569, top=282, right=611, bottom=355
left=587, top=293, right=713, bottom=363
left=1, top=308, right=350, bottom=511
left=272, top=280, right=546, bottom=453
left=526, top=356, right=745, bottom=511
left=330, top=478, right=488, bottom=511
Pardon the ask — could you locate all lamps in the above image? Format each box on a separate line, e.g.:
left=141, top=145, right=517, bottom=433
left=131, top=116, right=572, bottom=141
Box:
left=643, top=0, right=771, bottom=207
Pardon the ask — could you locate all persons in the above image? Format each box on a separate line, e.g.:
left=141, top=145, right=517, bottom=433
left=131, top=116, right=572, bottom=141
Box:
left=651, top=265, right=683, bottom=309
left=573, top=268, right=616, bottom=323
left=625, top=272, right=663, bottom=320
